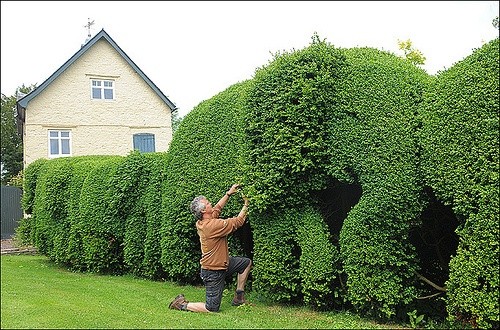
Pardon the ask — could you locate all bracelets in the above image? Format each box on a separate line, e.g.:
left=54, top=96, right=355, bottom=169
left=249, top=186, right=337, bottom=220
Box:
left=226, top=192, right=231, bottom=197
left=243, top=204, right=249, bottom=208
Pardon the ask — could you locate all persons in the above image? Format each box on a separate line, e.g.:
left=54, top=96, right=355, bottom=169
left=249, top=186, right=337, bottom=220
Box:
left=168, top=183, right=258, bottom=314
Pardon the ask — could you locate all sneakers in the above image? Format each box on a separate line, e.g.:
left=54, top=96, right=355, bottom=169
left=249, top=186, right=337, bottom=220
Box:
left=168, top=294, right=189, bottom=311
left=232, top=289, right=255, bottom=306
